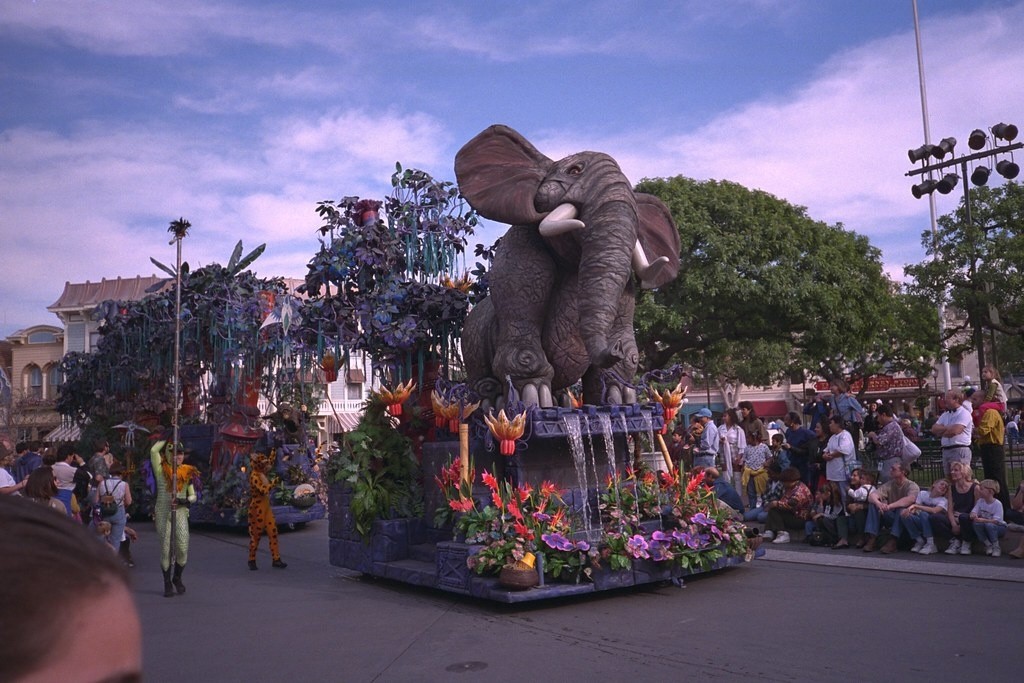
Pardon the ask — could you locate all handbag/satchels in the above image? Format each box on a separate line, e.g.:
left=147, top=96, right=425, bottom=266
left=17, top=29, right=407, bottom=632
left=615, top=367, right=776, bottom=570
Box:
left=843, top=420, right=852, bottom=432
left=844, top=459, right=862, bottom=482
left=902, top=435, right=922, bottom=466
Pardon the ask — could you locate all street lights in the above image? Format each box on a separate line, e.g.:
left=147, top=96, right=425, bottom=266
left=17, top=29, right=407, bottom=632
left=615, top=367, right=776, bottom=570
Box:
left=903, top=120, right=1024, bottom=373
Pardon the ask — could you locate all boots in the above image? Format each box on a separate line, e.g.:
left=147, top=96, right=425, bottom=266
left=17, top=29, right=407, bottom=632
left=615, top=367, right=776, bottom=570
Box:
left=161, top=564, right=174, bottom=598
left=172, top=562, right=185, bottom=593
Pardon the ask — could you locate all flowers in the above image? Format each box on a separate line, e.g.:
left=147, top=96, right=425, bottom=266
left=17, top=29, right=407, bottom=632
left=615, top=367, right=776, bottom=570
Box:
left=431, top=452, right=750, bottom=582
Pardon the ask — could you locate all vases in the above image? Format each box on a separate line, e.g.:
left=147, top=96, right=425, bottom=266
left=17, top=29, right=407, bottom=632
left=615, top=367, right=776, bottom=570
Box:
left=499, top=561, right=586, bottom=591
left=655, top=546, right=718, bottom=577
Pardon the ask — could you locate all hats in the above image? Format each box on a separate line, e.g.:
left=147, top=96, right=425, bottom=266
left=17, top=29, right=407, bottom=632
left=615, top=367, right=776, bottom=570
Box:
left=694, top=409, right=713, bottom=419
left=0, top=440, right=14, bottom=461
left=779, top=467, right=799, bottom=481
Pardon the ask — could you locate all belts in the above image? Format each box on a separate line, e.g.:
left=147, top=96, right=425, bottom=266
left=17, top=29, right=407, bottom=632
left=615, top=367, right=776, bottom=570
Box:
left=696, top=451, right=714, bottom=456
left=944, top=445, right=970, bottom=449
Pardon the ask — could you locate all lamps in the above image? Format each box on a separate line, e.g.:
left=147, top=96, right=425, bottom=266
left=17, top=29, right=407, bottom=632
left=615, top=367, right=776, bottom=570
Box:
left=904, top=122, right=1019, bottom=199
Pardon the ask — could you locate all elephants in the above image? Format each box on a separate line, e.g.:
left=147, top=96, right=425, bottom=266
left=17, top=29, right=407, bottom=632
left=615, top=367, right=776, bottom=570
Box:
left=454, top=124, right=681, bottom=424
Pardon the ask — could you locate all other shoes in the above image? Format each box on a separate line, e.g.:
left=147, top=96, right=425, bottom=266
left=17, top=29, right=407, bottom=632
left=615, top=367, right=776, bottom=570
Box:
left=273, top=559, right=288, bottom=568
left=1008, top=551, right=1024, bottom=559
left=772, top=531, right=790, bottom=544
left=763, top=531, right=776, bottom=541
left=247, top=560, right=258, bottom=570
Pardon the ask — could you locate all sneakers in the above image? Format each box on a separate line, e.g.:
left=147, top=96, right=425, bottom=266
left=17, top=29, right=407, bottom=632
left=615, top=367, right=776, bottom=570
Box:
left=992, top=546, right=1002, bottom=557
left=944, top=538, right=963, bottom=554
left=984, top=545, right=992, bottom=555
left=801, top=536, right=938, bottom=554
left=960, top=541, right=972, bottom=555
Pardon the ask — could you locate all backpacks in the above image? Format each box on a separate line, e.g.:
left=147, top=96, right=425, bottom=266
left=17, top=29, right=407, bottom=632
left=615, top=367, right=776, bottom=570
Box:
left=99, top=479, right=122, bottom=517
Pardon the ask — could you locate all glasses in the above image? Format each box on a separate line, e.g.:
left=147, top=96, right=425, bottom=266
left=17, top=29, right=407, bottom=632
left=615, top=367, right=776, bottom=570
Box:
left=54, top=475, right=57, bottom=480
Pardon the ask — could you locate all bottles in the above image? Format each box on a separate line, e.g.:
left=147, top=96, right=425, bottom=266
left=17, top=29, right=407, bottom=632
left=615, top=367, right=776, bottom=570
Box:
left=93, top=509, right=101, bottom=525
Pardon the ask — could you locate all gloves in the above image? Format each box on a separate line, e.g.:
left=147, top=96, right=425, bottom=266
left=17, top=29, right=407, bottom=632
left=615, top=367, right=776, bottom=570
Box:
left=175, top=496, right=189, bottom=507
left=159, top=428, right=173, bottom=441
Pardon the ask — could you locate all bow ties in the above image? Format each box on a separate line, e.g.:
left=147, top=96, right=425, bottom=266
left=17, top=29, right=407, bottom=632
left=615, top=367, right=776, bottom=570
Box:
left=949, top=409, right=956, bottom=413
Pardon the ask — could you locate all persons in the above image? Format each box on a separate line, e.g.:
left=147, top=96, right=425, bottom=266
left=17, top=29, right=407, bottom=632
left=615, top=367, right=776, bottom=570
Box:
left=0, top=494, right=146, bottom=683
left=670, top=362, right=1024, bottom=558
left=150, top=415, right=197, bottom=598
left=0, top=438, right=138, bottom=566
left=249, top=446, right=287, bottom=570
